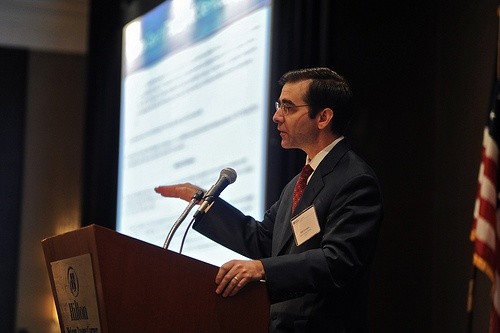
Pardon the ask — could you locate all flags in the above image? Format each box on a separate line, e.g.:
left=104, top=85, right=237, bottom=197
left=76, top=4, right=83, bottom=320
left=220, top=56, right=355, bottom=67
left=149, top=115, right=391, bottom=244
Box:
left=467, top=99, right=500, bottom=333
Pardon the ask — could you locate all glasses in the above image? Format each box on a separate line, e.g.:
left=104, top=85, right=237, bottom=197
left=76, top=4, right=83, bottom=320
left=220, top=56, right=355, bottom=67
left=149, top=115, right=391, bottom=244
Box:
left=275, top=100, right=313, bottom=115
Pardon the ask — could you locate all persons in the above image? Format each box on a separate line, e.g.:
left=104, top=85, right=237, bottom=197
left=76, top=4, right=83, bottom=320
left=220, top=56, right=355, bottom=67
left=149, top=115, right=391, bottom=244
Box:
left=154, top=67, right=381, bottom=332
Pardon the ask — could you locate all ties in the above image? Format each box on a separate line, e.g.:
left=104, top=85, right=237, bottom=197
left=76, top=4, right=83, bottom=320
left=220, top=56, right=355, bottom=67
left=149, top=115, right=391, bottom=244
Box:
left=291, top=164, right=315, bottom=214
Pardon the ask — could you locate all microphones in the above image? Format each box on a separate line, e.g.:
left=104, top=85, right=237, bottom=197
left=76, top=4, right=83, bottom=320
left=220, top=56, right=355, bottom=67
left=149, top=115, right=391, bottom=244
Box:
left=162, top=190, right=204, bottom=249
left=193, top=167, right=237, bottom=219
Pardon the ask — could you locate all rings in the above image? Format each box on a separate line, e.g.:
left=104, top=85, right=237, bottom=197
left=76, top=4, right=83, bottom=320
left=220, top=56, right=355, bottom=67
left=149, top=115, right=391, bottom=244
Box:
left=234, top=277, right=239, bottom=282
